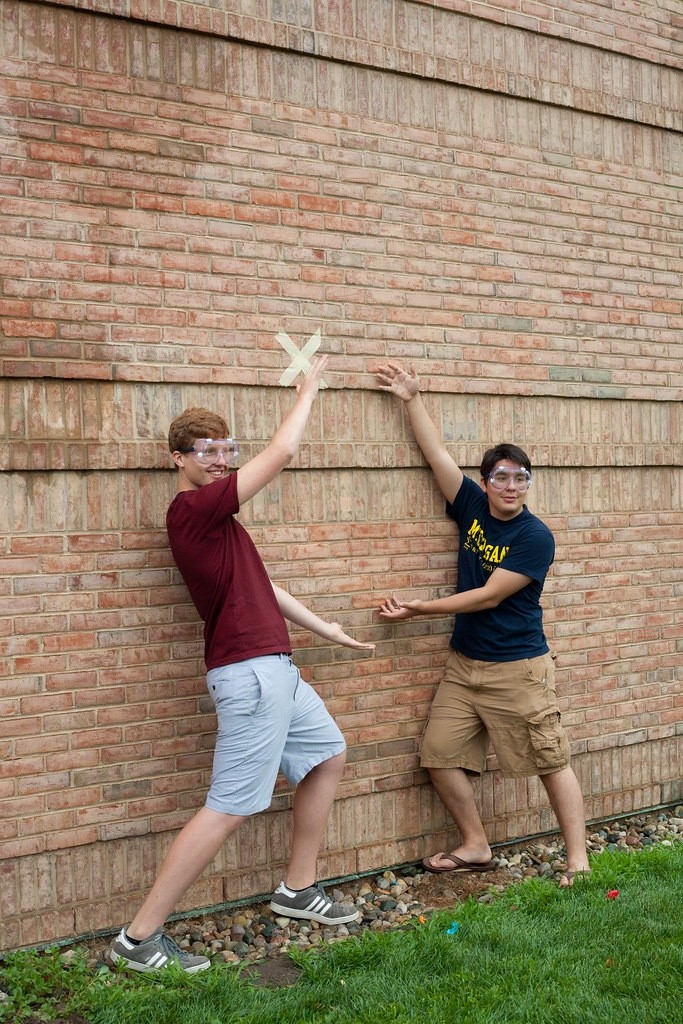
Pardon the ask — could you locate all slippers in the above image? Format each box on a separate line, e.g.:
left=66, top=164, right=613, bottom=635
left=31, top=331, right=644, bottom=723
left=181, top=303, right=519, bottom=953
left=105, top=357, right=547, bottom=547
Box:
left=557, top=869, right=591, bottom=889
left=422, top=852, right=496, bottom=871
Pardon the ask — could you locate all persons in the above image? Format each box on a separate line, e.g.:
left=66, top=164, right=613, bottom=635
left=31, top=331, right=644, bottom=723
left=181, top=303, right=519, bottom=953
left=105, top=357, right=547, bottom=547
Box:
left=376, top=362, right=590, bottom=889
left=110, top=354, right=375, bottom=976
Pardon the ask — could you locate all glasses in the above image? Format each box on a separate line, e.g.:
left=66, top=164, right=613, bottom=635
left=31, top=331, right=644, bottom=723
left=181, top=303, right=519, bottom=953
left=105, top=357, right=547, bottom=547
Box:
left=485, top=465, right=532, bottom=493
left=192, top=439, right=239, bottom=467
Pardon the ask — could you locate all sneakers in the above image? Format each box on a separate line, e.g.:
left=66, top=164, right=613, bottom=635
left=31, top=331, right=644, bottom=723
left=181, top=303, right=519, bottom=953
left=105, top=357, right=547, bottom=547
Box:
left=269, top=880, right=360, bottom=925
left=111, top=927, right=211, bottom=977
